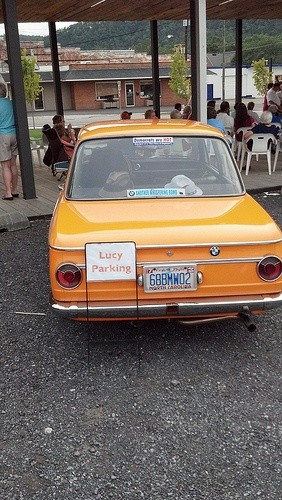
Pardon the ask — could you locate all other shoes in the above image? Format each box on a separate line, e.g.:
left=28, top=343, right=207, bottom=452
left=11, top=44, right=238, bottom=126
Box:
left=2, top=197, right=14, bottom=200
left=11, top=193, right=19, bottom=197
left=242, top=167, right=251, bottom=171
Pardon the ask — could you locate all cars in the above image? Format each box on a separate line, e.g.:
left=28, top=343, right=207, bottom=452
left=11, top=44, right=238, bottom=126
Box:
left=48, top=120, right=281, bottom=332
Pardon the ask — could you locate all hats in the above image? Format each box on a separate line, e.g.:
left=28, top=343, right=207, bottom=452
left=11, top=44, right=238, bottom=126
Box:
left=98, top=171, right=134, bottom=198
left=164, top=175, right=203, bottom=196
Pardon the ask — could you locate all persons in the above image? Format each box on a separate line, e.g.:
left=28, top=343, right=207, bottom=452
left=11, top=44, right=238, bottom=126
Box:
left=217, top=101, right=234, bottom=130
left=52, top=115, right=72, bottom=134
left=207, top=100, right=216, bottom=109
left=231, top=102, right=259, bottom=142
left=0, top=83, right=19, bottom=201
left=263, top=82, right=282, bottom=111
left=268, top=104, right=282, bottom=123
left=121, top=110, right=132, bottom=120
left=241, top=111, right=277, bottom=171
left=145, top=109, right=158, bottom=119
left=54, top=124, right=77, bottom=160
left=207, top=107, right=226, bottom=134
left=170, top=103, right=193, bottom=119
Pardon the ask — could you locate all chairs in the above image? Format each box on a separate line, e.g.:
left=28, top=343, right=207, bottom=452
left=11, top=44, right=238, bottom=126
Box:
left=87, top=148, right=134, bottom=192
left=224, top=122, right=282, bottom=175
left=30, top=124, right=71, bottom=182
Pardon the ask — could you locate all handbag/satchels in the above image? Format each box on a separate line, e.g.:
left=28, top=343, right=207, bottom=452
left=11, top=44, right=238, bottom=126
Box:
left=43, top=145, right=51, bottom=166
